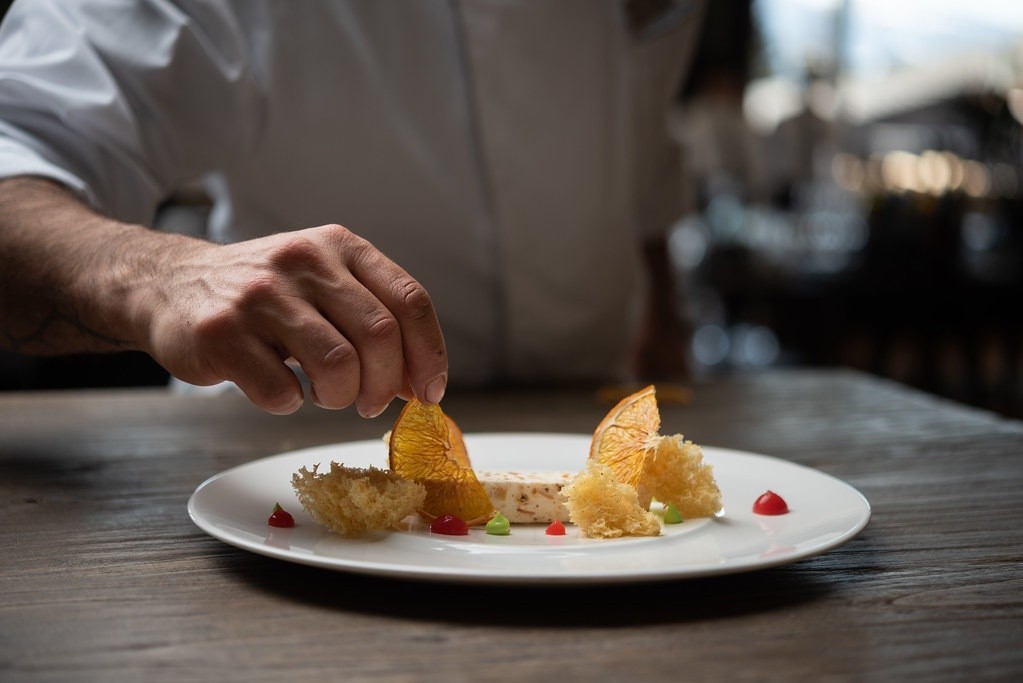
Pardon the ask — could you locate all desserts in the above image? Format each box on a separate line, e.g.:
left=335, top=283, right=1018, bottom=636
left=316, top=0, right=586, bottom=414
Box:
left=473, top=469, right=571, bottom=525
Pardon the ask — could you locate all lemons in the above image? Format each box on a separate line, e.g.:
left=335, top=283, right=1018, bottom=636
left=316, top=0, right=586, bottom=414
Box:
left=590, top=385, right=662, bottom=492
left=389, top=395, right=495, bottom=525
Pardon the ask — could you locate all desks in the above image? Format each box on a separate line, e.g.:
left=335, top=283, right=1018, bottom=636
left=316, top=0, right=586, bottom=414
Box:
left=0, top=370, right=1023, bottom=683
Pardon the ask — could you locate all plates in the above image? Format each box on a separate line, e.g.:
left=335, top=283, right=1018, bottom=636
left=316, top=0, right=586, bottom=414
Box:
left=186, top=434, right=872, bottom=582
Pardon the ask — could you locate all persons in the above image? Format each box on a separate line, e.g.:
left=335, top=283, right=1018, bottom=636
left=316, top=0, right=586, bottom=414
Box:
left=1, top=0, right=868, bottom=421
